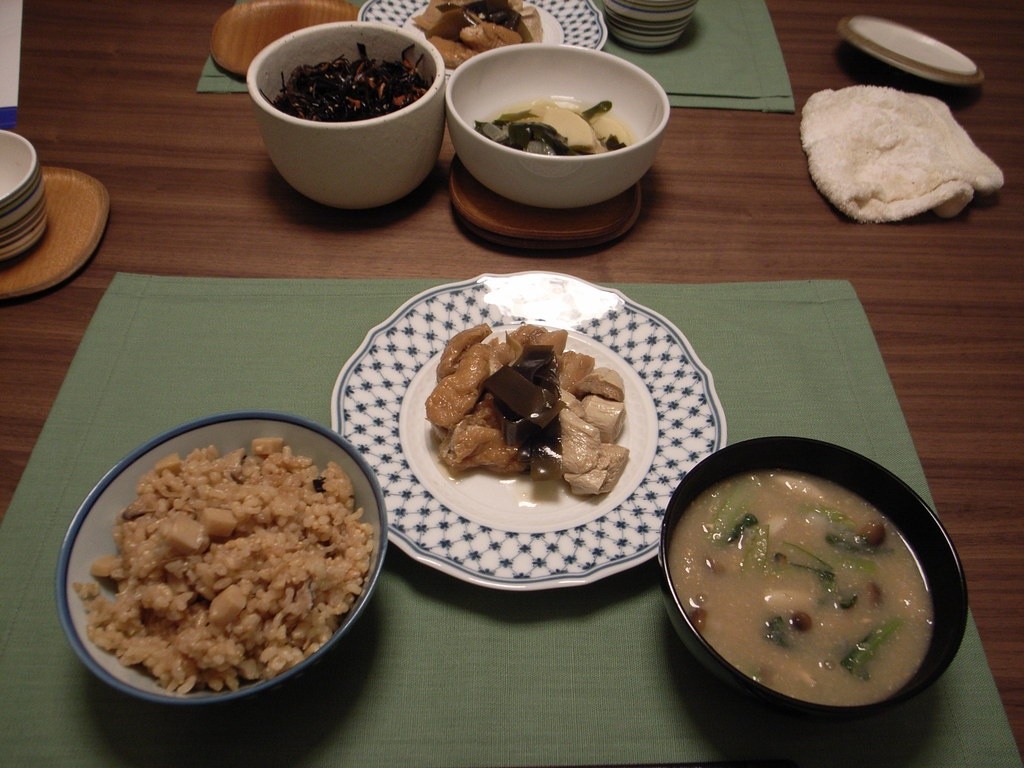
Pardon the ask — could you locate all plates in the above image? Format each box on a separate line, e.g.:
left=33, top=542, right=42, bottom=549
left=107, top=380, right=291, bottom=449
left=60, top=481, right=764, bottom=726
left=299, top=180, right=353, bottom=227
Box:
left=330, top=271, right=727, bottom=591
left=356, top=0, right=607, bottom=91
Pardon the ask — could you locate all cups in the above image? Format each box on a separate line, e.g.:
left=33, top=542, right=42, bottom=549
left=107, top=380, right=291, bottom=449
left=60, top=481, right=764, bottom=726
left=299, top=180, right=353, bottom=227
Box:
left=0, top=129, right=49, bottom=272
left=603, top=0, right=698, bottom=50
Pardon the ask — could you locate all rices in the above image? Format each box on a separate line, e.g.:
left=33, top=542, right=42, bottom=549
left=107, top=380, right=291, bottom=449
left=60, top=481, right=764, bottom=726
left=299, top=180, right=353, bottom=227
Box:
left=71, top=439, right=376, bottom=697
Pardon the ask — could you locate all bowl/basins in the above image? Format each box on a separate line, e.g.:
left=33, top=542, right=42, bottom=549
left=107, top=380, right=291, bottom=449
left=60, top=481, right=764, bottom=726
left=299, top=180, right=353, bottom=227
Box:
left=247, top=20, right=446, bottom=209
left=446, top=43, right=670, bottom=208
left=658, top=436, right=968, bottom=719
left=55, top=411, right=388, bottom=704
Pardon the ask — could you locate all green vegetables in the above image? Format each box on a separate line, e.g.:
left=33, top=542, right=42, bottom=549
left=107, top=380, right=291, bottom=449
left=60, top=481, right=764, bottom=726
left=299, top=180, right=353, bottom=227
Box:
left=706, top=485, right=905, bottom=681
